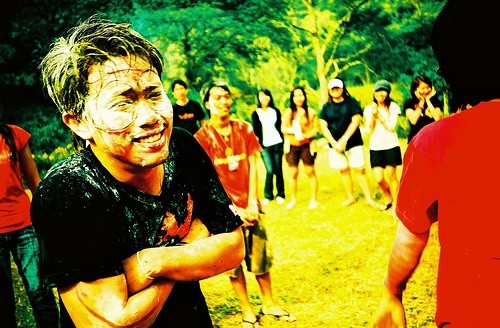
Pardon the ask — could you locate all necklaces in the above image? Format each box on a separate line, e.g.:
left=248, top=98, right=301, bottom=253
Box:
left=210, top=118, right=235, bottom=157
left=217, top=129, right=230, bottom=141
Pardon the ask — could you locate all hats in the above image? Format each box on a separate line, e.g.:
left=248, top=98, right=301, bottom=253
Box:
left=328, top=78, right=343, bottom=89
left=375, top=80, right=391, bottom=92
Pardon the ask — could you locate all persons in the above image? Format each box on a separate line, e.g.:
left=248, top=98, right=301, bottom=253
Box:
left=0, top=74, right=443, bottom=328
left=373, top=0, right=499, bottom=328
left=27, top=12, right=247, bottom=328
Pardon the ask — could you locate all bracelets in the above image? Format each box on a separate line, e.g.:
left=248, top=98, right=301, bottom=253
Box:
left=136, top=251, right=156, bottom=282
left=419, top=106, right=424, bottom=110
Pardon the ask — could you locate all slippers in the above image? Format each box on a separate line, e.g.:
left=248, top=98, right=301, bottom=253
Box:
left=242, top=319, right=261, bottom=328
left=262, top=309, right=296, bottom=323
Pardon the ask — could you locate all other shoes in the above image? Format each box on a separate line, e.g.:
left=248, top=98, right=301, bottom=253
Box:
left=367, top=199, right=376, bottom=206
left=344, top=200, right=355, bottom=205
left=262, top=198, right=271, bottom=205
left=276, top=196, right=285, bottom=204
left=385, top=203, right=392, bottom=210
left=287, top=201, right=296, bottom=208
left=310, top=202, right=317, bottom=209
